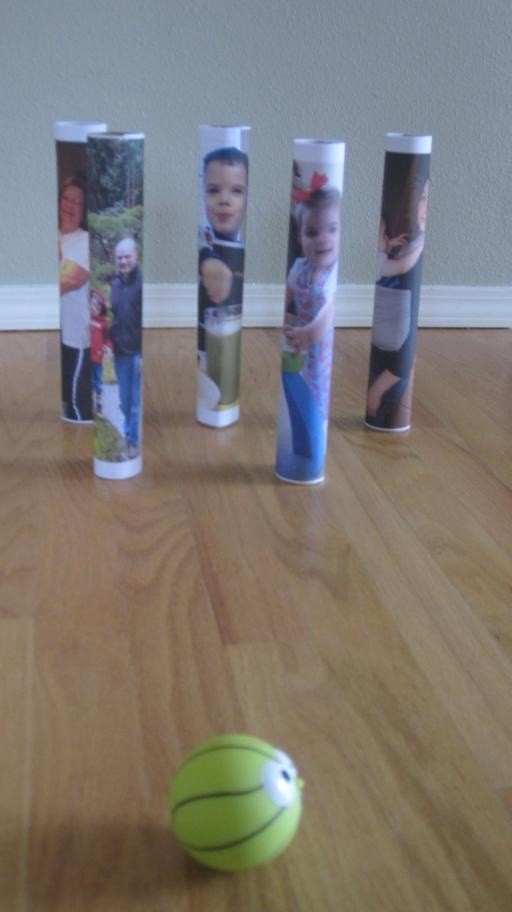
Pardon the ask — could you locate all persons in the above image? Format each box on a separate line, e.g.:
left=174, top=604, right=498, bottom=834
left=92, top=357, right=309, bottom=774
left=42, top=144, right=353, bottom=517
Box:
left=110, top=236, right=141, bottom=460
left=84, top=285, right=115, bottom=417
left=283, top=187, right=342, bottom=481
left=197, top=148, right=249, bottom=305
left=364, top=176, right=430, bottom=429
left=374, top=216, right=409, bottom=282
left=56, top=178, right=97, bottom=420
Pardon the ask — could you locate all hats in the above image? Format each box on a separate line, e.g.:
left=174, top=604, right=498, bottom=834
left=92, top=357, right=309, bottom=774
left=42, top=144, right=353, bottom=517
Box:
left=92, top=288, right=106, bottom=315
